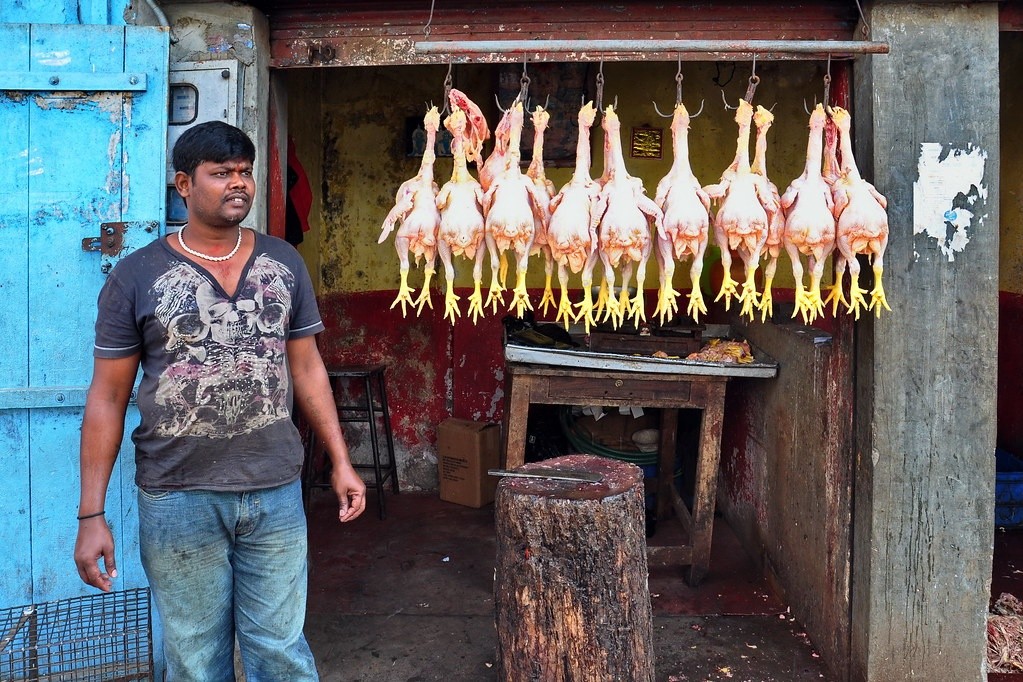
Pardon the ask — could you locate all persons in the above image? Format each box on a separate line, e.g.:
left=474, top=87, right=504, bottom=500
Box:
left=75, top=120, right=370, bottom=682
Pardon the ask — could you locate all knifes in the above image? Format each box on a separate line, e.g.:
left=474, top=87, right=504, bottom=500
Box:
left=487, top=467, right=602, bottom=482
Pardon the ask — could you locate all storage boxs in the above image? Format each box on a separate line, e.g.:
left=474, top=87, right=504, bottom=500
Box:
left=438, top=418, right=500, bottom=508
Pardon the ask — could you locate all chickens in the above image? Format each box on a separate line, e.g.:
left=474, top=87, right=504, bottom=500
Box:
left=377, top=105, right=894, bottom=336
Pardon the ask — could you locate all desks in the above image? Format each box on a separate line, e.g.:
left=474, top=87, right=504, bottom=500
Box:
left=503, top=363, right=731, bottom=589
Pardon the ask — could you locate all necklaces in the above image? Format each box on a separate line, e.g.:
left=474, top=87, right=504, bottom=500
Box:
left=178, top=221, right=243, bottom=263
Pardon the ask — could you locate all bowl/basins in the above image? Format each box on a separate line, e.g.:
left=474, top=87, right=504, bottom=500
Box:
left=580, top=285, right=637, bottom=317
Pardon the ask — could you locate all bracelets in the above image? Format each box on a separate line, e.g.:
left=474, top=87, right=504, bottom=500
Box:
left=76, top=511, right=106, bottom=520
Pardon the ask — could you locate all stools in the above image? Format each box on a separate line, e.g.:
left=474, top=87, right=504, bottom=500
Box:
left=304, top=364, right=399, bottom=522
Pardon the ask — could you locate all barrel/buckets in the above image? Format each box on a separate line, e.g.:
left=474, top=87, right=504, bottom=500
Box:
left=560, top=406, right=680, bottom=543
left=996, top=450, right=1023, bottom=524
